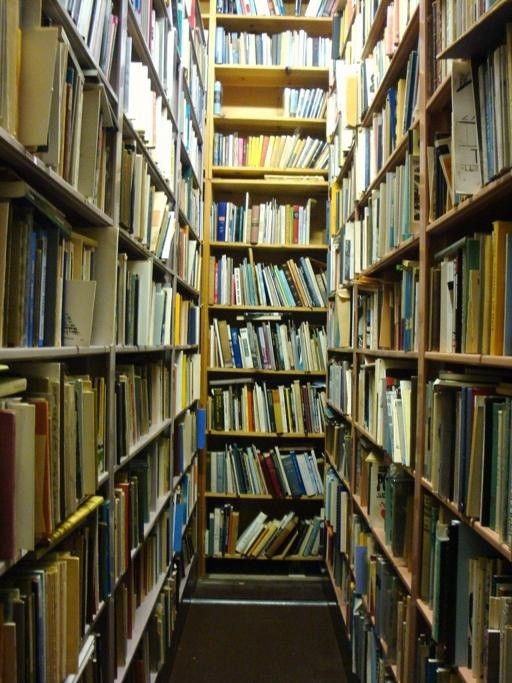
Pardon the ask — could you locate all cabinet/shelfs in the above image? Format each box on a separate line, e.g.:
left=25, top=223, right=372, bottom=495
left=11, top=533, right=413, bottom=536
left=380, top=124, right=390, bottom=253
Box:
left=198, top=1, right=333, bottom=581
left=0, top=0, right=209, bottom=683
left=323, top=0, right=511, bottom=682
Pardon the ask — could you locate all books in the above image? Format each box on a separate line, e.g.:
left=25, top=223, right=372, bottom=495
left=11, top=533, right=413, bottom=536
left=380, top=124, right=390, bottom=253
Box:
left=0, top=1, right=203, bottom=683
left=203, top=1, right=336, bottom=560
left=329, top=0, right=512, bottom=682
